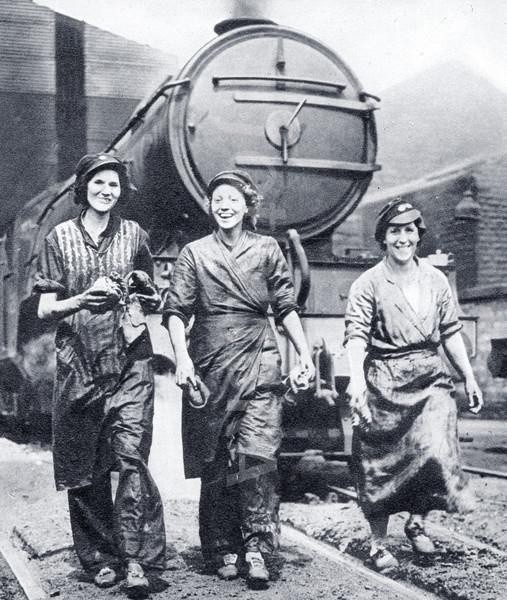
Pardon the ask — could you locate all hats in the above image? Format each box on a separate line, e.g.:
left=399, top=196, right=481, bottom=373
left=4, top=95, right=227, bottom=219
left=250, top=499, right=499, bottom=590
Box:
left=377, top=198, right=427, bottom=236
left=76, top=152, right=123, bottom=176
left=208, top=170, right=256, bottom=193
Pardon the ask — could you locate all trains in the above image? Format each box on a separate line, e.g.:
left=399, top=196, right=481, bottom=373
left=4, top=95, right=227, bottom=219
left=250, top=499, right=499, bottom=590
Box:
left=0, top=1, right=481, bottom=498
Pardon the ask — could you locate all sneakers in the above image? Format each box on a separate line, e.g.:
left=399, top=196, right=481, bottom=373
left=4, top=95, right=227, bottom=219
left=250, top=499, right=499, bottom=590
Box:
left=406, top=521, right=433, bottom=552
left=245, top=551, right=269, bottom=581
left=219, top=552, right=240, bottom=578
left=370, top=547, right=394, bottom=568
left=94, top=568, right=115, bottom=587
left=126, top=563, right=149, bottom=590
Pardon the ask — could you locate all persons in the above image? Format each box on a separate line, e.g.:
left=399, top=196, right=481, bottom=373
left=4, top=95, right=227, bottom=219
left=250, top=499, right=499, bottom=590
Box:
left=343, top=198, right=484, bottom=575
left=160, top=170, right=319, bottom=584
left=32, top=152, right=167, bottom=594
